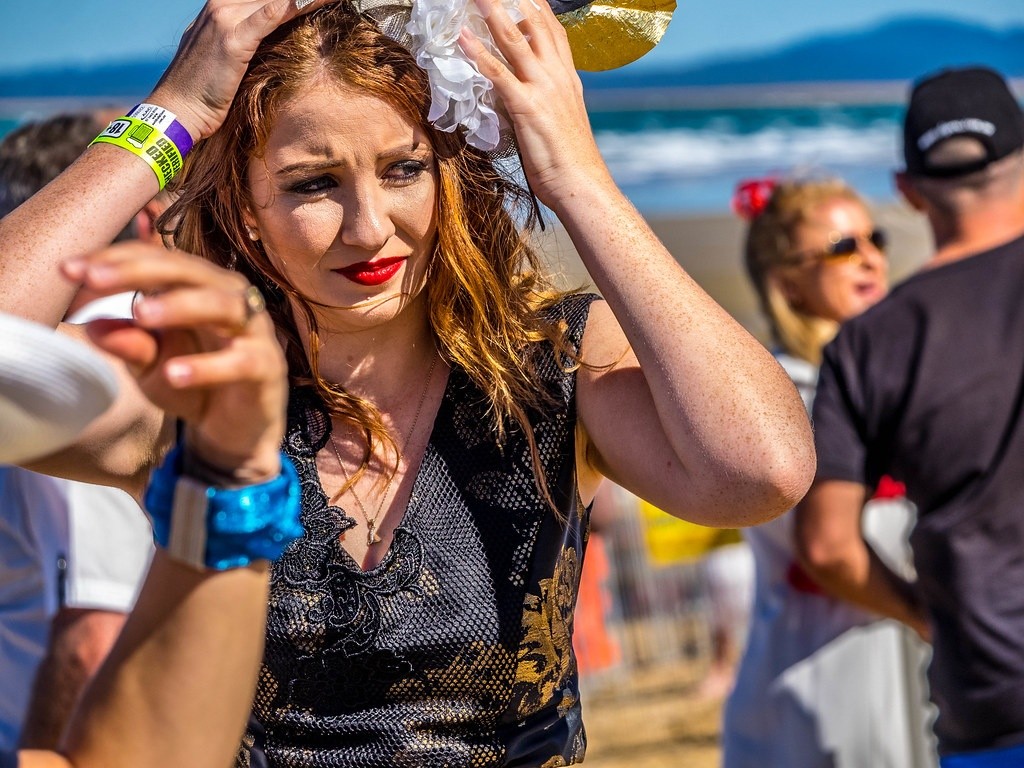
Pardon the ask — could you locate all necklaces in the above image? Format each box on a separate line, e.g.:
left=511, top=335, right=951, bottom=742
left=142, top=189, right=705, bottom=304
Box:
left=327, top=340, right=440, bottom=545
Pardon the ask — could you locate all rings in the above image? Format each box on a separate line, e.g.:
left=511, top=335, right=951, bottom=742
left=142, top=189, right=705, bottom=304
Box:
left=241, top=284, right=269, bottom=336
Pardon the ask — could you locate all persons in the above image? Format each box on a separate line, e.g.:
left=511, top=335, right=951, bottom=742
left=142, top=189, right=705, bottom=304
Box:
left=0, top=1, right=819, bottom=768
left=790, top=64, right=1024, bottom=768
left=0, top=230, right=294, bottom=768
left=0, top=116, right=185, bottom=255
left=719, top=178, right=940, bottom=768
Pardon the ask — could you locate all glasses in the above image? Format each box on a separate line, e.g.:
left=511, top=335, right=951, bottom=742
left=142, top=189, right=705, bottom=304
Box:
left=781, top=231, right=887, bottom=267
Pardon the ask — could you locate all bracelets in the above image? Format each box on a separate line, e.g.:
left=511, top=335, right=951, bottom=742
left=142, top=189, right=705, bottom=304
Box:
left=132, top=438, right=307, bottom=580
left=83, top=100, right=199, bottom=194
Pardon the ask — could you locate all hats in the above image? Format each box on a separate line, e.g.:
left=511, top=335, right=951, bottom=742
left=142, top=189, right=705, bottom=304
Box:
left=904, top=68, right=1024, bottom=181
